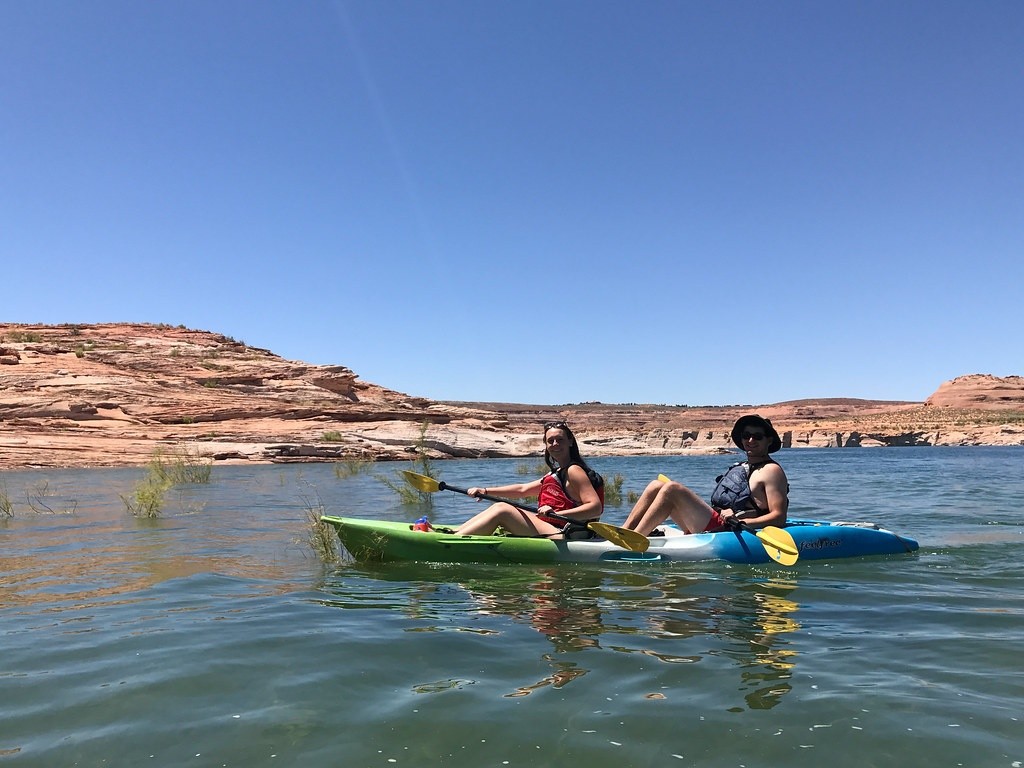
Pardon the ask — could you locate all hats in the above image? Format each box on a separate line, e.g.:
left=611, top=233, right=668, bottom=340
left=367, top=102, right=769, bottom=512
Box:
left=730, top=413, right=781, bottom=454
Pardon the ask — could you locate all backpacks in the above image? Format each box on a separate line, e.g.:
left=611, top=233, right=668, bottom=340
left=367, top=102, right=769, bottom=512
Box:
left=711, top=461, right=761, bottom=511
left=561, top=462, right=605, bottom=517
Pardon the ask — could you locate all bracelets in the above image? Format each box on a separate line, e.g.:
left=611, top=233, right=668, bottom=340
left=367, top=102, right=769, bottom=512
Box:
left=484, top=488, right=487, bottom=495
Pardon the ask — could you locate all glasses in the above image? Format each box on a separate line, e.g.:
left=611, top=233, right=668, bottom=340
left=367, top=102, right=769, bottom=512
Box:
left=741, top=430, right=766, bottom=441
left=543, top=421, right=567, bottom=430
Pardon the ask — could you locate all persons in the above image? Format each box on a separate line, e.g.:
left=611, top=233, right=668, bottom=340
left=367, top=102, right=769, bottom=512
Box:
left=448, top=422, right=603, bottom=540
left=728, top=568, right=799, bottom=710
left=621, top=413, right=789, bottom=538
left=528, top=566, right=605, bottom=690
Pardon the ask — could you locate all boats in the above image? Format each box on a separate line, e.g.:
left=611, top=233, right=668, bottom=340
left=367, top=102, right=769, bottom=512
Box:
left=319, top=513, right=921, bottom=563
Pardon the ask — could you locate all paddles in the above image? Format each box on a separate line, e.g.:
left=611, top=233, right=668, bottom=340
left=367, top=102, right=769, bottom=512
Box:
left=657, top=472, right=800, bottom=566
left=403, top=470, right=650, bottom=553
